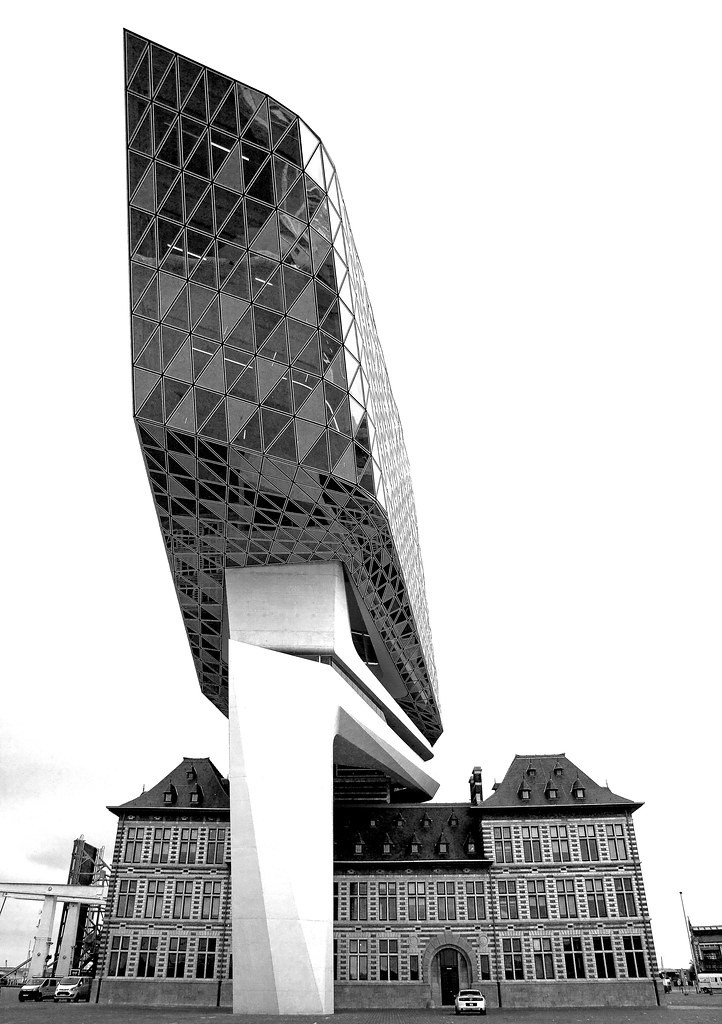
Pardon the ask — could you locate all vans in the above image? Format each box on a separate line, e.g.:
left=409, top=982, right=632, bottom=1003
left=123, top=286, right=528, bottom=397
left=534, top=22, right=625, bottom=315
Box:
left=18, top=976, right=62, bottom=1002
left=54, top=976, right=93, bottom=1002
left=697, top=973, right=722, bottom=993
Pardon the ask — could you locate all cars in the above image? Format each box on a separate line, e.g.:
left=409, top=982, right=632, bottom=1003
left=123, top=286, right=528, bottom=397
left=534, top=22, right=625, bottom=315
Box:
left=454, top=989, right=486, bottom=1015
